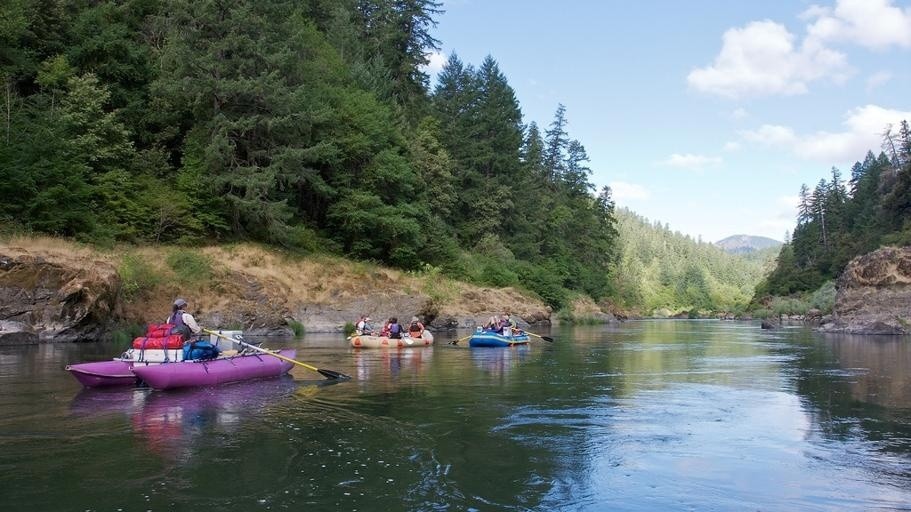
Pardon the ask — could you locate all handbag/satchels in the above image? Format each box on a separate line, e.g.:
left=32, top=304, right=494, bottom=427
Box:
left=183, top=340, right=220, bottom=360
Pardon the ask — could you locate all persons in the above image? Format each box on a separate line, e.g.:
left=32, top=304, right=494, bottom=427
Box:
left=355, top=314, right=425, bottom=339
left=483, top=314, right=522, bottom=337
left=167, top=298, right=206, bottom=343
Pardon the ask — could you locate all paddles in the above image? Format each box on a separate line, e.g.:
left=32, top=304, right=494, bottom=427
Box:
left=202, top=328, right=352, bottom=381
left=449, top=335, right=471, bottom=345
left=511, top=326, right=555, bottom=343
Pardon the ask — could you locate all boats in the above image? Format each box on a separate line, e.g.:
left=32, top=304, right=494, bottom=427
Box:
left=348, top=328, right=435, bottom=348
left=64, top=347, right=298, bottom=393
left=467, top=325, right=531, bottom=348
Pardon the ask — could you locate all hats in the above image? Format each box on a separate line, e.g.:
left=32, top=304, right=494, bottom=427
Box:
left=173, top=299, right=188, bottom=309
